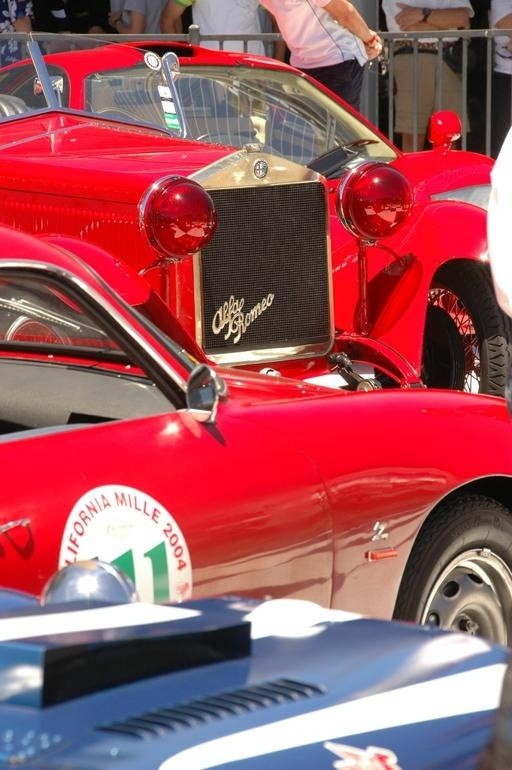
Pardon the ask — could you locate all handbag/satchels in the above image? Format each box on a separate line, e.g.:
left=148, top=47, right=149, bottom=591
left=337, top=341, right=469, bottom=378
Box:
left=443, top=44, right=462, bottom=73
left=378, top=56, right=397, bottom=99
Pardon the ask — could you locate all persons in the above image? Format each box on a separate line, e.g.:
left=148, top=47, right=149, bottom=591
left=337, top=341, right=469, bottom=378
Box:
left=0, top=0, right=512, bottom=150
left=485, top=127, right=512, bottom=320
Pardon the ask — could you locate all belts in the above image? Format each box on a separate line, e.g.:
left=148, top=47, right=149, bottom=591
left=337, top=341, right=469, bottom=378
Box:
left=393, top=47, right=439, bottom=56
